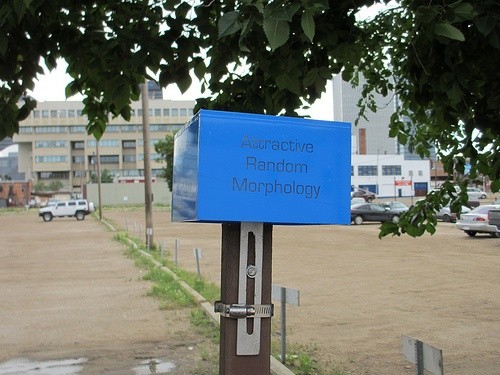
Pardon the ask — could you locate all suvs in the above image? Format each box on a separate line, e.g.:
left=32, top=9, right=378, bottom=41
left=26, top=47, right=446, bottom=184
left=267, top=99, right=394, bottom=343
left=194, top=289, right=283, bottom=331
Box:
left=39, top=198, right=95, bottom=222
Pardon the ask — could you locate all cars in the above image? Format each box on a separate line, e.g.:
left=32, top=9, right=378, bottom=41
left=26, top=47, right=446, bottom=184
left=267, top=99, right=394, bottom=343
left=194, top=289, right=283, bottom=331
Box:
left=28, top=196, right=41, bottom=208
left=456, top=205, right=500, bottom=238
left=419, top=185, right=472, bottom=223
left=466, top=186, right=487, bottom=199
left=350, top=186, right=410, bottom=225
left=45, top=198, right=59, bottom=207
